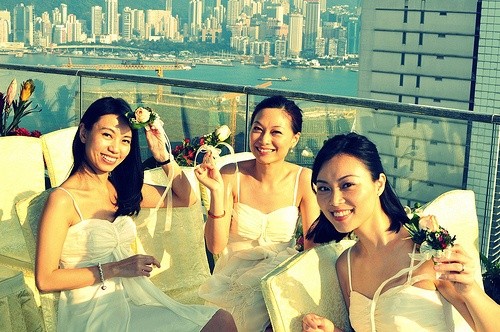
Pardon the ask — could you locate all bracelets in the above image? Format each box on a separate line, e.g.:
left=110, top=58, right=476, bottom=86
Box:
left=97, top=263, right=107, bottom=290
left=157, top=159, right=171, bottom=166
left=207, top=210, right=226, bottom=219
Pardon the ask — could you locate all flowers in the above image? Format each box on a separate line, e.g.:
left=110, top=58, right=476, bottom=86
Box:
left=172, top=132, right=217, bottom=168
left=402, top=211, right=457, bottom=279
left=207, top=124, right=232, bottom=158
left=0, top=78, right=43, bottom=138
left=123, top=104, right=165, bottom=135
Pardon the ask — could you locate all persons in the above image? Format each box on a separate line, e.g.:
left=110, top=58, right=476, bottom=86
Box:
left=303, top=134, right=500, bottom=332
left=36, top=97, right=238, bottom=332
left=194, top=96, right=320, bottom=332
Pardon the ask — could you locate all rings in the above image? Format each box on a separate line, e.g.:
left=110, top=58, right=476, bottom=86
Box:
left=459, top=263, right=465, bottom=273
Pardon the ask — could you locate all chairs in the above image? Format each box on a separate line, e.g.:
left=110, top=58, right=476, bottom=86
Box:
left=0, top=126, right=486, bottom=332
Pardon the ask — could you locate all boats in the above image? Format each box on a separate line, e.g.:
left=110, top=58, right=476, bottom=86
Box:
left=120, top=53, right=144, bottom=65
left=257, top=76, right=292, bottom=82
